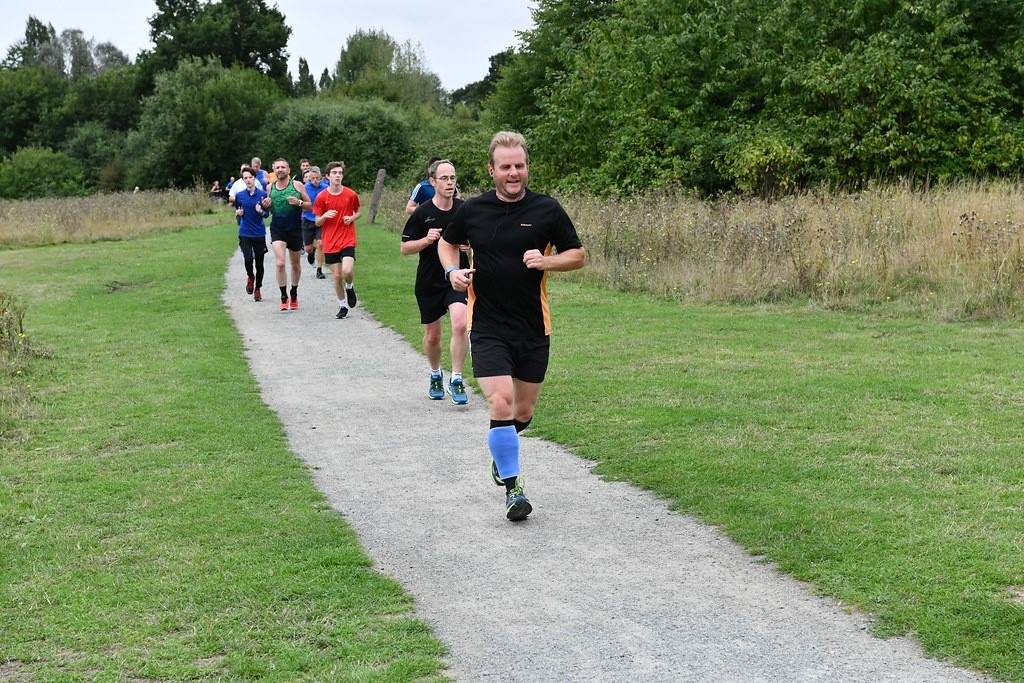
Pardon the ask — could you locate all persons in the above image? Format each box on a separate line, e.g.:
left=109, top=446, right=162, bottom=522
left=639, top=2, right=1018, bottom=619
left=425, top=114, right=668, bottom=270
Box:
left=261, top=158, right=312, bottom=311
left=312, top=162, right=361, bottom=318
left=134, top=186, right=139, bottom=194
left=229, top=163, right=263, bottom=202
left=438, top=131, right=586, bottom=522
left=252, top=157, right=269, bottom=189
left=226, top=177, right=234, bottom=206
left=292, top=158, right=330, bottom=279
left=234, top=167, right=269, bottom=302
left=211, top=181, right=222, bottom=199
left=400, top=160, right=470, bottom=405
left=406, top=157, right=460, bottom=214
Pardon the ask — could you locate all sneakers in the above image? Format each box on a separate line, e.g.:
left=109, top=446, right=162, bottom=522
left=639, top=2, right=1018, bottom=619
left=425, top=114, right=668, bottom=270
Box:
left=429, top=369, right=444, bottom=400
left=505, top=476, right=532, bottom=520
left=280, top=296, right=289, bottom=310
left=246, top=276, right=255, bottom=294
left=290, top=291, right=298, bottom=309
left=335, top=307, right=348, bottom=319
left=308, top=248, right=315, bottom=264
left=447, top=377, right=469, bottom=405
left=490, top=458, right=505, bottom=486
left=254, top=288, right=261, bottom=301
left=317, top=272, right=325, bottom=278
left=346, top=286, right=357, bottom=308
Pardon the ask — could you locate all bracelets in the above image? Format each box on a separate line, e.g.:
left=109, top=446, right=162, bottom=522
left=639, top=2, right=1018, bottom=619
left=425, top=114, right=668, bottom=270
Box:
left=445, top=266, right=458, bottom=280
left=299, top=200, right=303, bottom=206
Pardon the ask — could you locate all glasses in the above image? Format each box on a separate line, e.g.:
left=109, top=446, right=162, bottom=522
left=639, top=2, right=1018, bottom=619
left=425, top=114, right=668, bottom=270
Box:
left=437, top=176, right=457, bottom=182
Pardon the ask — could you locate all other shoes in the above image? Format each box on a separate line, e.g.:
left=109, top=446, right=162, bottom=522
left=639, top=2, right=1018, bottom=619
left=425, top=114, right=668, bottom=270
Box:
left=301, top=246, right=305, bottom=255
